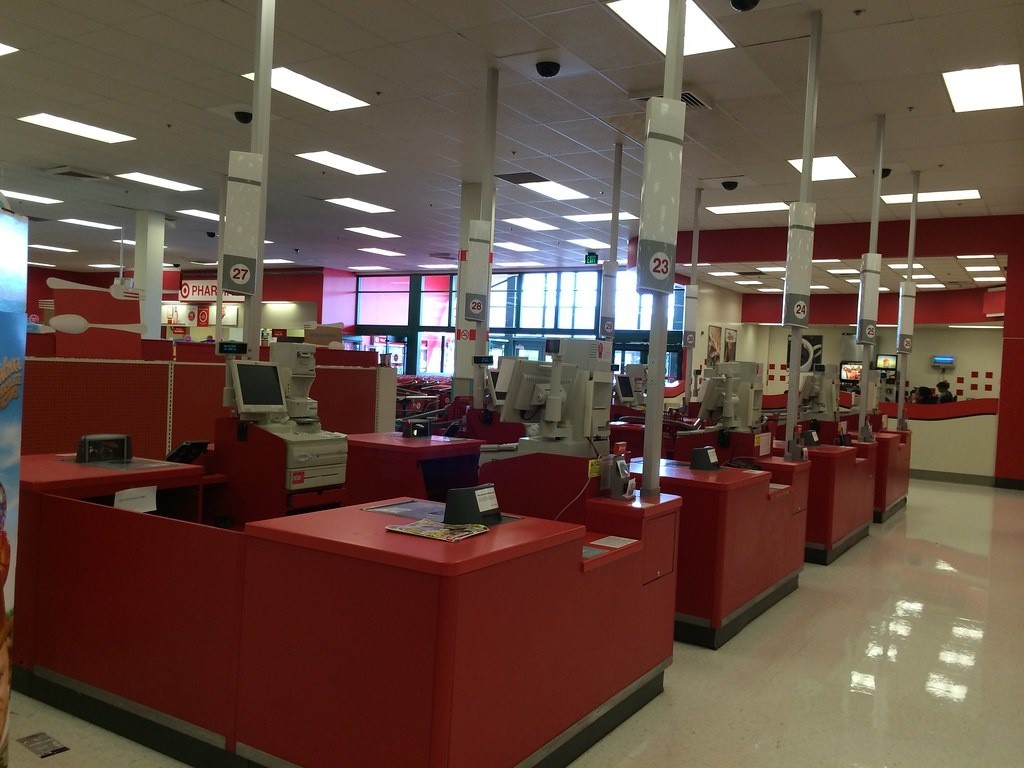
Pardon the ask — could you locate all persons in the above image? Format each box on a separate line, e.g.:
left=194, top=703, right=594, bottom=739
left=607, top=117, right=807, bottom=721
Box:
left=936, top=380, right=954, bottom=404
left=914, top=386, right=938, bottom=404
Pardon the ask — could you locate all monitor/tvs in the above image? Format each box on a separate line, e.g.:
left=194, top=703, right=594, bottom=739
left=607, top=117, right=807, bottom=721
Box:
left=230, top=360, right=288, bottom=413
left=797, top=375, right=821, bottom=406
left=615, top=374, right=636, bottom=401
left=499, top=360, right=580, bottom=423
left=839, top=361, right=863, bottom=383
left=876, top=354, right=898, bottom=370
left=487, top=368, right=507, bottom=406
left=703, top=377, right=729, bottom=411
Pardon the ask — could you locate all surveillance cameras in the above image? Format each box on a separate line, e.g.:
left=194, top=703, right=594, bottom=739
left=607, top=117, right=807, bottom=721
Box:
left=174, top=264, right=180, bottom=267
left=206, top=231, right=216, bottom=238
left=536, top=62, right=560, bottom=78
left=234, top=112, right=253, bottom=124
left=872, top=168, right=891, bottom=179
left=730, top=0, right=759, bottom=12
left=722, top=181, right=738, bottom=190
left=295, top=249, right=300, bottom=252
